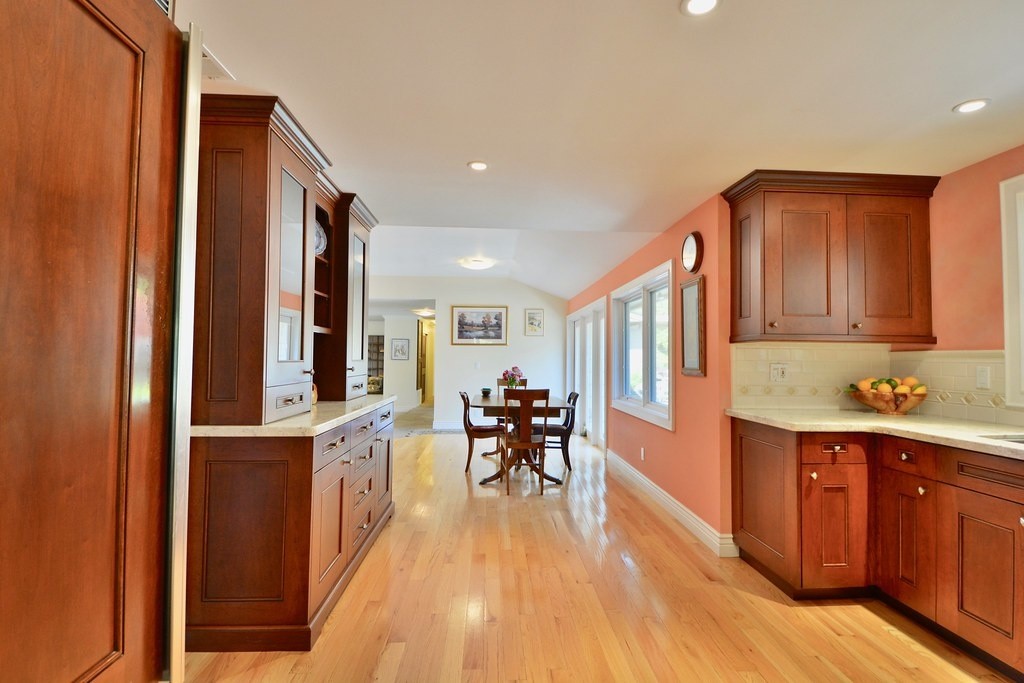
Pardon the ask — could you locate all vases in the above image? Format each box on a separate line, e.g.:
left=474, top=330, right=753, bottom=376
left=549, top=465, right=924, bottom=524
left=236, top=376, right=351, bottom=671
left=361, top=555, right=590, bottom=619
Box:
left=509, top=385, right=516, bottom=389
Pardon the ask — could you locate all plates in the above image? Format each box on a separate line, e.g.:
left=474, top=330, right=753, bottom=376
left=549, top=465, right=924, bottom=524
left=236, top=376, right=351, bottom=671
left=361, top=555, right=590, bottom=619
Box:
left=315, top=219, right=327, bottom=256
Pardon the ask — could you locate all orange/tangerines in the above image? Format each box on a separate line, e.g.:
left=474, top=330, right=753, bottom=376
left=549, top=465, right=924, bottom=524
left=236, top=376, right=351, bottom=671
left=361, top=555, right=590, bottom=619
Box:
left=855, top=376, right=928, bottom=393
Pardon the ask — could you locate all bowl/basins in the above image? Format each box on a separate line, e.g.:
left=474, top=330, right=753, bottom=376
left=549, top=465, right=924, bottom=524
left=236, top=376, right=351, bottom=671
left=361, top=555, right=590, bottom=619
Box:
left=848, top=391, right=928, bottom=416
left=481, top=389, right=491, bottom=395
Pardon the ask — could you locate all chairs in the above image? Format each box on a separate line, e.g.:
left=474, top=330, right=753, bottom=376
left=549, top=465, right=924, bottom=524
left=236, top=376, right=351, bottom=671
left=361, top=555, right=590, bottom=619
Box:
left=460, top=379, right=579, bottom=495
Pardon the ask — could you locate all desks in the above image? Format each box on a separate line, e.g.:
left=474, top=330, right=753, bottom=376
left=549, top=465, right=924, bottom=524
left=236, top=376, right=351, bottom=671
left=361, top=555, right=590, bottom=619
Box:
left=471, top=393, right=575, bottom=485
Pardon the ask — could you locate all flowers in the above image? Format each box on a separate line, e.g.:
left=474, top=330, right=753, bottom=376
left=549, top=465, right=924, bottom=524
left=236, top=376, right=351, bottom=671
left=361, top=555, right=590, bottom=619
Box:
left=503, top=366, right=524, bottom=386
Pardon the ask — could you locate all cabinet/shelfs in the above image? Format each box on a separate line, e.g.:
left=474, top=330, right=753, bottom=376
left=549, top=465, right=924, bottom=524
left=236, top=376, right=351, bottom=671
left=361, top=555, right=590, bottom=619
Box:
left=720, top=167, right=941, bottom=344
left=731, top=417, right=1024, bottom=675
left=191, top=93, right=380, bottom=425
left=185, top=395, right=396, bottom=653
left=0, top=0, right=202, bottom=683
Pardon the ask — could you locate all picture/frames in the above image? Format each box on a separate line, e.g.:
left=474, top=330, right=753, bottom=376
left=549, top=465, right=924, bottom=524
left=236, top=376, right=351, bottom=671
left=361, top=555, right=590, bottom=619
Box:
left=451, top=305, right=508, bottom=345
left=525, top=309, right=545, bottom=336
left=680, top=274, right=706, bottom=376
left=391, top=338, right=409, bottom=360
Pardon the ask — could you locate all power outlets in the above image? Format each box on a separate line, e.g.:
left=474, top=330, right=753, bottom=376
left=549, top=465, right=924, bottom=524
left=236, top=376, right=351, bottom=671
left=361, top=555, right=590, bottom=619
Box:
left=769, top=363, right=789, bottom=382
